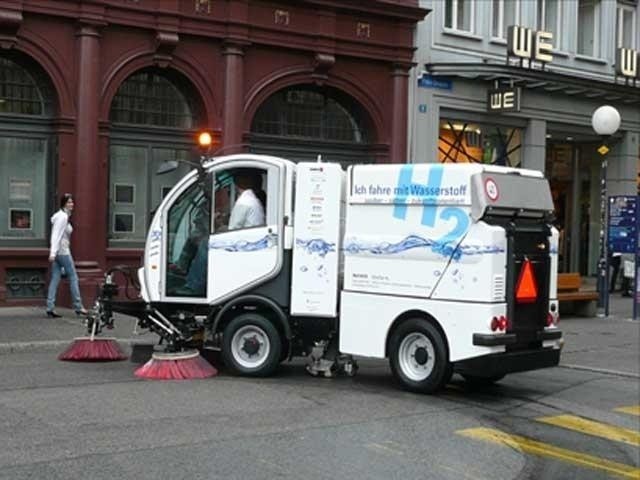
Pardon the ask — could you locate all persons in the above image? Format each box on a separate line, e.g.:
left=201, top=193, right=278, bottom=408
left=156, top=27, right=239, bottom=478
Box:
left=174, top=169, right=265, bottom=295
left=168, top=174, right=266, bottom=275
left=596, top=210, right=634, bottom=309
left=15, top=213, right=28, bottom=228
left=45, top=192, right=90, bottom=319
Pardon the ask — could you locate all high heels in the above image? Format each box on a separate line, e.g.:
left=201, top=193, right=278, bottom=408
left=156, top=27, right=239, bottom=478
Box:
left=75, top=310, right=90, bottom=318
left=46, top=311, right=63, bottom=319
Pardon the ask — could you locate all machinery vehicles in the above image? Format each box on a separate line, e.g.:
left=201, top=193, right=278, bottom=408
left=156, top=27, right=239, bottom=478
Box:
left=52, top=130, right=570, bottom=399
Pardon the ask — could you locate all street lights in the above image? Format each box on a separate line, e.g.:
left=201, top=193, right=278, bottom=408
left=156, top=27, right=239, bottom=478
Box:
left=590, top=102, right=622, bottom=318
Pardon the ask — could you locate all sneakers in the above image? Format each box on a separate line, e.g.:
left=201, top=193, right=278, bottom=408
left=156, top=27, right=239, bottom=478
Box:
left=168, top=268, right=189, bottom=281
left=176, top=287, right=204, bottom=297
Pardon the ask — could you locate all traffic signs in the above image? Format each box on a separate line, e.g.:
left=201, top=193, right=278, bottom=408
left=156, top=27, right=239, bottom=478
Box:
left=608, top=195, right=639, bottom=254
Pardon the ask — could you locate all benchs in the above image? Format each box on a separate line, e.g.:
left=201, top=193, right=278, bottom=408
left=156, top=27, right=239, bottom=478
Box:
left=555, top=273, right=599, bottom=303
